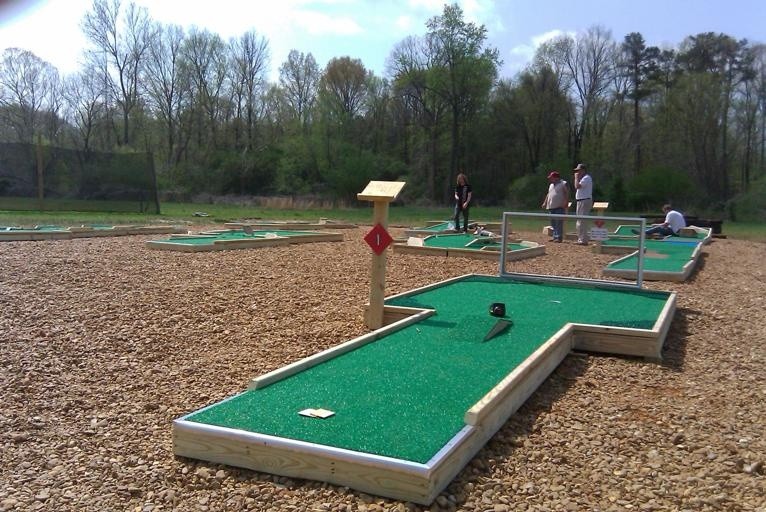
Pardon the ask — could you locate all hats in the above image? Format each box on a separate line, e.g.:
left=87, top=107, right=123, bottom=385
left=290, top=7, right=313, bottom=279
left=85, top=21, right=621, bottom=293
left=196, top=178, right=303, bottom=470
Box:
left=574, top=164, right=587, bottom=171
left=546, top=171, right=561, bottom=179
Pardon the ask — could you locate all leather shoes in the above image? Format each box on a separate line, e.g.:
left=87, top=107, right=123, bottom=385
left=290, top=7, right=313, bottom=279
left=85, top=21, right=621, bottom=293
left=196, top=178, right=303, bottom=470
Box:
left=572, top=240, right=587, bottom=245
left=632, top=228, right=640, bottom=234
left=453, top=230, right=467, bottom=233
left=549, top=238, right=561, bottom=243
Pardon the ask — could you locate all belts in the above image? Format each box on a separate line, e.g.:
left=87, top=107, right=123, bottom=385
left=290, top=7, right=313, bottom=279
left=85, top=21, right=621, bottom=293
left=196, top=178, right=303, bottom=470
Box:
left=550, top=207, right=564, bottom=210
left=577, top=198, right=590, bottom=201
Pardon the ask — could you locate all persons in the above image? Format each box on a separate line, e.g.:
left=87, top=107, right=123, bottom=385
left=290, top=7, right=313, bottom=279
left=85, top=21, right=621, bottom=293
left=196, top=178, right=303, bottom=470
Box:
left=631, top=204, right=686, bottom=236
left=542, top=164, right=593, bottom=245
left=454, top=173, right=472, bottom=234
